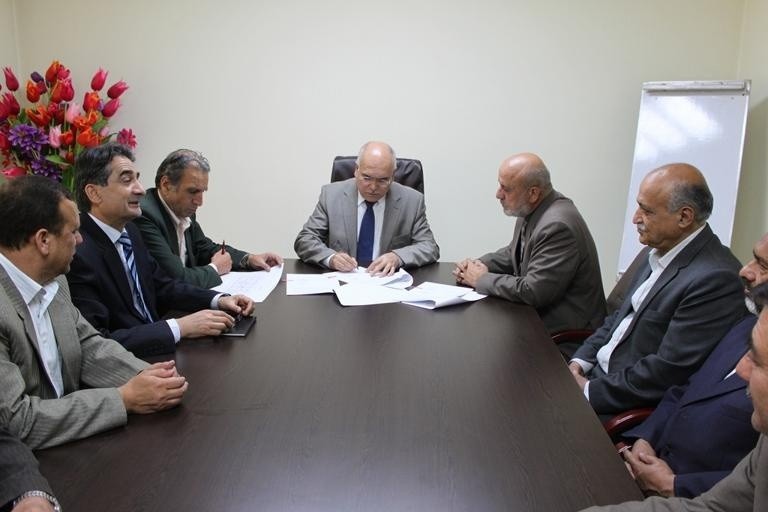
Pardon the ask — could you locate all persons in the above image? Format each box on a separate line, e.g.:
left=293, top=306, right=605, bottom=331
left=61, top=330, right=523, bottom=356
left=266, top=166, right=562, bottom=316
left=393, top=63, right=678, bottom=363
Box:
left=64, top=141, right=255, bottom=356
left=617, top=232, right=768, bottom=497
left=0, top=174, right=189, bottom=452
left=451, top=152, right=608, bottom=362
left=294, top=140, right=440, bottom=278
left=0, top=429, right=62, bottom=511
left=569, top=162, right=749, bottom=440
left=135, top=147, right=284, bottom=289
left=578, top=303, right=768, bottom=511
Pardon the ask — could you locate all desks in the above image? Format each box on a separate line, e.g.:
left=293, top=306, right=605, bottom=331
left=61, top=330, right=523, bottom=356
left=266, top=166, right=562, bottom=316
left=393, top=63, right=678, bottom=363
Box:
left=26, top=251, right=648, bottom=512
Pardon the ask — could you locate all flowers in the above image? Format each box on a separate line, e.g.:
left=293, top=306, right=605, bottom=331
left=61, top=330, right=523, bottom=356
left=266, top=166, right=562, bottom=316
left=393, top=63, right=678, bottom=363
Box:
left=1, top=60, right=138, bottom=206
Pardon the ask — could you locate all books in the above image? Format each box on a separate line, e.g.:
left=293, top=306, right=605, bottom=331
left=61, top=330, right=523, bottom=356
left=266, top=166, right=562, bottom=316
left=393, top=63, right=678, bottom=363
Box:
left=162, top=309, right=257, bottom=338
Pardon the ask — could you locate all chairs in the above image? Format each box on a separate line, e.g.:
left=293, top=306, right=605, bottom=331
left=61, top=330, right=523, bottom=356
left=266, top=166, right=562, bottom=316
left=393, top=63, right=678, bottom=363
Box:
left=331, top=155, right=423, bottom=195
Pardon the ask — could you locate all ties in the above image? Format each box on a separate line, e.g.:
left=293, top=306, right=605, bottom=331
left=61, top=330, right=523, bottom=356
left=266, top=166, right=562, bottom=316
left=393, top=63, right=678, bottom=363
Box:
left=117, top=233, right=153, bottom=322
left=357, top=200, right=377, bottom=267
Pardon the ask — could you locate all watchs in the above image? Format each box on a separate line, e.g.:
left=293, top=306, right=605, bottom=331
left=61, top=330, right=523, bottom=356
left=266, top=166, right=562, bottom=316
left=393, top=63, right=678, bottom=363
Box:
left=12, top=490, right=62, bottom=512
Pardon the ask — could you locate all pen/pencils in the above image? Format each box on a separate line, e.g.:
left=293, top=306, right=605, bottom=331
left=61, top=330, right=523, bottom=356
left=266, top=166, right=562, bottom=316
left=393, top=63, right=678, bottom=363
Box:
left=346, top=240, right=359, bottom=270
left=221, top=240, right=224, bottom=254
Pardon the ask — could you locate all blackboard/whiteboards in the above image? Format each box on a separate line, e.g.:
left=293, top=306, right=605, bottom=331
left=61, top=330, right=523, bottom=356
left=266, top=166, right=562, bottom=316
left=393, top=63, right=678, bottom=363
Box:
left=615, top=79, right=752, bottom=283
